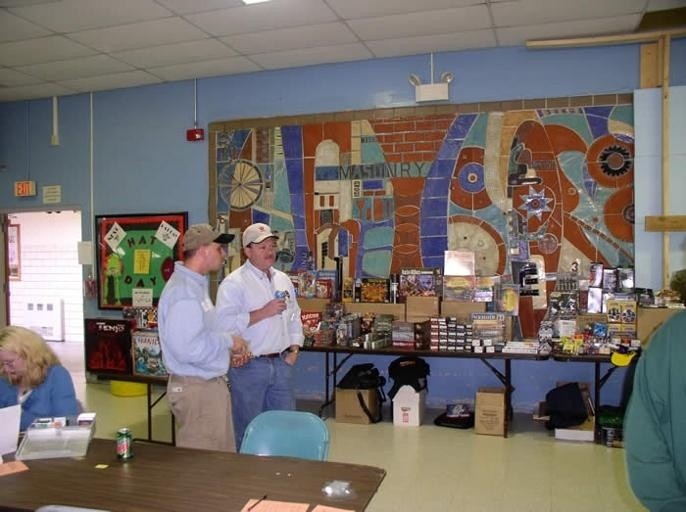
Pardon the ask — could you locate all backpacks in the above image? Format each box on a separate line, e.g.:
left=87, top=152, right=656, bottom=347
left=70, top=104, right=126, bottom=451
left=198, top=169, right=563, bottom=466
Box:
left=387, top=356, right=431, bottom=400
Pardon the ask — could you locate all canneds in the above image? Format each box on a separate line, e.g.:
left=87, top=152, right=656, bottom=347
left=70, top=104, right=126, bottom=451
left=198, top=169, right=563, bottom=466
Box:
left=115, top=428, right=132, bottom=461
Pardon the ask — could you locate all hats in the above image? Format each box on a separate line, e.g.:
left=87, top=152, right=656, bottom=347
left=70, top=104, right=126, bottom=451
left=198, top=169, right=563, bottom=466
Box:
left=242, top=223, right=280, bottom=249
left=182, top=224, right=235, bottom=253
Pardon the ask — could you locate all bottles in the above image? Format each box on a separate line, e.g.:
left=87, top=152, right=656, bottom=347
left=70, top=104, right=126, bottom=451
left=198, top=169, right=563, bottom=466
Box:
left=390, top=272, right=399, bottom=304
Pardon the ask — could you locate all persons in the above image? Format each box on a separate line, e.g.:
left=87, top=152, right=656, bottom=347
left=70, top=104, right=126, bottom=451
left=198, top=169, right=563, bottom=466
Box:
left=0, top=326, right=77, bottom=433
left=624, top=269, right=686, bottom=511
left=157, top=223, right=252, bottom=454
left=215, top=223, right=305, bottom=455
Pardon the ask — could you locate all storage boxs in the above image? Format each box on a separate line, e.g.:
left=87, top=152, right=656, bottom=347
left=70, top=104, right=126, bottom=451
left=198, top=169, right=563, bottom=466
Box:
left=335, top=386, right=378, bottom=425
left=637, top=305, right=680, bottom=350
left=441, top=301, right=486, bottom=323
left=297, top=297, right=330, bottom=312
left=406, top=297, right=439, bottom=323
left=393, top=378, right=427, bottom=427
left=345, top=303, right=405, bottom=322
left=475, top=387, right=505, bottom=435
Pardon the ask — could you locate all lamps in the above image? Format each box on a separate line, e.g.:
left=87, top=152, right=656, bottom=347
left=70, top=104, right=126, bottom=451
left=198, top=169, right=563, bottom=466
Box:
left=407, top=54, right=454, bottom=103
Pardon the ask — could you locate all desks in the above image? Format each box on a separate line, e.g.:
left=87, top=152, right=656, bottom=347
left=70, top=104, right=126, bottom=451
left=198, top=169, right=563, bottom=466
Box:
left=0, top=433, right=386, bottom=512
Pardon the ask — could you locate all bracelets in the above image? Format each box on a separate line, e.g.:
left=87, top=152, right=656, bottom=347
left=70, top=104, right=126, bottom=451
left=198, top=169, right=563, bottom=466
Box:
left=290, top=350, right=300, bottom=353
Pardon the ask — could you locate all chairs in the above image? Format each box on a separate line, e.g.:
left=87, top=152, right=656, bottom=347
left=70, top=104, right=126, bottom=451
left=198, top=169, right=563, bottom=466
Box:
left=239, top=409, right=329, bottom=464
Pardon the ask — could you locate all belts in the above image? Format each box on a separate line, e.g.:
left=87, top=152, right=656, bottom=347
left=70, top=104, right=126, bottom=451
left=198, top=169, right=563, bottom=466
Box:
left=261, top=351, right=285, bottom=359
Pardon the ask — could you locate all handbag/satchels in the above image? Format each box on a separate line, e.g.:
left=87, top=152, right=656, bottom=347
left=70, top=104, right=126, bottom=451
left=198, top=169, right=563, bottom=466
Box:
left=336, top=362, right=387, bottom=424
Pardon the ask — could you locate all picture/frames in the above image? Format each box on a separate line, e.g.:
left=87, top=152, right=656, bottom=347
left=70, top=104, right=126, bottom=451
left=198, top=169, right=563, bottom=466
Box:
left=94, top=211, right=188, bottom=310
left=7, top=224, right=21, bottom=281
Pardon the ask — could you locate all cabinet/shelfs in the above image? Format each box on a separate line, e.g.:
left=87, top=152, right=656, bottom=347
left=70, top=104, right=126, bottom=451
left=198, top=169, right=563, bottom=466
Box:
left=551, top=349, right=641, bottom=444
left=129, top=373, right=175, bottom=445
left=299, top=346, right=551, bottom=439
